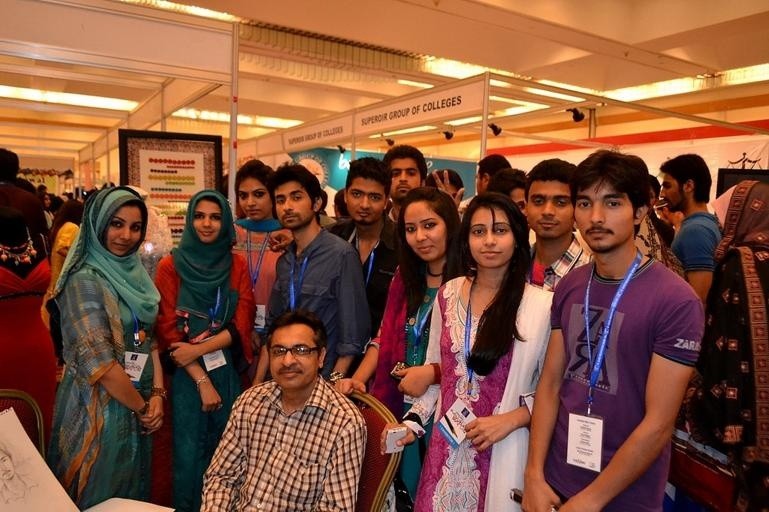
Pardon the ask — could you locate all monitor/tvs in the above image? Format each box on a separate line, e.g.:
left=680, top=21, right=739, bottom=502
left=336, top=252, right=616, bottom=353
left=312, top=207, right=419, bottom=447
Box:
left=715, top=167, right=769, bottom=200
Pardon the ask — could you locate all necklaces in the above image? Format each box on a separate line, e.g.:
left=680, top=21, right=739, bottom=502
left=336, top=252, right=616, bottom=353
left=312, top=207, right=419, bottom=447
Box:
left=0, top=226, right=37, bottom=266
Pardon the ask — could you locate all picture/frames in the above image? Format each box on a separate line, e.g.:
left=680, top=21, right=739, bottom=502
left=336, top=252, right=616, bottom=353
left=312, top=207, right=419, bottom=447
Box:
left=117, top=127, right=224, bottom=251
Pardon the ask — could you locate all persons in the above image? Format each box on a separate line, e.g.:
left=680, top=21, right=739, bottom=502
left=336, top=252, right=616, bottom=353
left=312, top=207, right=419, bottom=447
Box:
left=271, top=157, right=399, bottom=341
left=656, top=200, right=685, bottom=232
left=230, top=159, right=285, bottom=378
left=648, top=177, right=674, bottom=245
left=46, top=185, right=167, bottom=511
left=382, top=145, right=428, bottom=222
left=153, top=190, right=256, bottom=512
left=684, top=181, right=769, bottom=472
left=200, top=309, right=367, bottom=512
left=425, top=169, right=464, bottom=207
left=0, top=148, right=48, bottom=239
left=41, top=200, right=82, bottom=334
left=476, top=155, right=512, bottom=194
left=0, top=444, right=38, bottom=504
left=658, top=153, right=723, bottom=314
left=334, top=186, right=469, bottom=512
left=38, top=184, right=54, bottom=229
left=522, top=159, right=594, bottom=291
left=432, top=169, right=538, bottom=259
left=380, top=192, right=554, bottom=511
left=252, top=163, right=370, bottom=387
left=522, top=150, right=704, bottom=512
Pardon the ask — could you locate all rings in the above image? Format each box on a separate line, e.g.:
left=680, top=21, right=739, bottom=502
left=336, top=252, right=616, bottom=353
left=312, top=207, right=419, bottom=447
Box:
left=217, top=404, right=223, bottom=410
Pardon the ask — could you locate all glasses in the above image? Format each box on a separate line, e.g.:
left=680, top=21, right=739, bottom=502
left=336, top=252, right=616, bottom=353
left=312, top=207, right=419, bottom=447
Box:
left=270, top=345, right=318, bottom=357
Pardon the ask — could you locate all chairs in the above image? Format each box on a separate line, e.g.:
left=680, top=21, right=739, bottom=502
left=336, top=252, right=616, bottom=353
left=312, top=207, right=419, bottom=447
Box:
left=0, top=387, right=45, bottom=460
left=347, top=391, right=402, bottom=512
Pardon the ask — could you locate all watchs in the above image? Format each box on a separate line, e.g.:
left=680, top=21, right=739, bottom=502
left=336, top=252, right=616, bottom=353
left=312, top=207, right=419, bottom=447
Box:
left=151, top=386, right=167, bottom=400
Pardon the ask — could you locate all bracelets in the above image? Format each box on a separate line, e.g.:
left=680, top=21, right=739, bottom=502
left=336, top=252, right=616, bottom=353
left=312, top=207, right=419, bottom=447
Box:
left=196, top=375, right=210, bottom=384
left=135, top=402, right=151, bottom=417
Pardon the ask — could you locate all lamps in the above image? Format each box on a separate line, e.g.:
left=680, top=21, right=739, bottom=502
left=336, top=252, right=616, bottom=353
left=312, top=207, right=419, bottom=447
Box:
left=386, top=139, right=394, bottom=146
left=567, top=108, right=584, bottom=123
left=489, top=124, right=502, bottom=136
left=337, top=145, right=346, bottom=153
left=444, top=131, right=454, bottom=141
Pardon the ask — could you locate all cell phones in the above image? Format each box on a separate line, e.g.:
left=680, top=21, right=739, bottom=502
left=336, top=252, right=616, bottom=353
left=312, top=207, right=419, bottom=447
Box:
left=383, top=428, right=407, bottom=454
